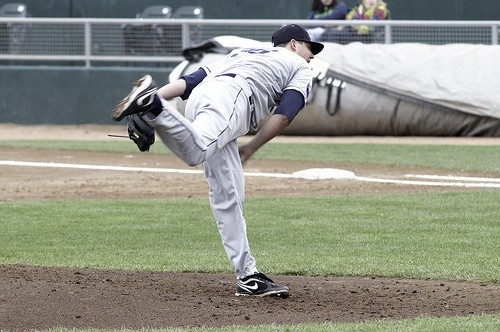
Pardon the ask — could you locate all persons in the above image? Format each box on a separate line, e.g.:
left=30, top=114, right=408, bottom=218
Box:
left=330, top=0, right=388, bottom=44
left=307, top=0, right=346, bottom=43
left=107, top=24, right=324, bottom=298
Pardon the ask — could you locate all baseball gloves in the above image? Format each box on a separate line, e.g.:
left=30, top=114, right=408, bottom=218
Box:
left=108, top=111, right=154, bottom=153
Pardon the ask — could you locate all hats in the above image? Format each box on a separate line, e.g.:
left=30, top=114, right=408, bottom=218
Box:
left=272, top=24, right=324, bottom=55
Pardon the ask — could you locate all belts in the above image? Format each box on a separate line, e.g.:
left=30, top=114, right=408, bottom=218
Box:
left=249, top=96, right=253, bottom=124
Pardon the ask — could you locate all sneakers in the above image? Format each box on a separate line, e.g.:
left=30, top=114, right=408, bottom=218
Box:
left=235, top=272, right=290, bottom=298
left=112, top=74, right=158, bottom=122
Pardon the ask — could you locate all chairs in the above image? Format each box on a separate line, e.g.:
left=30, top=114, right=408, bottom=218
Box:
left=122, top=6, right=204, bottom=55
left=2, top=4, right=27, bottom=51
